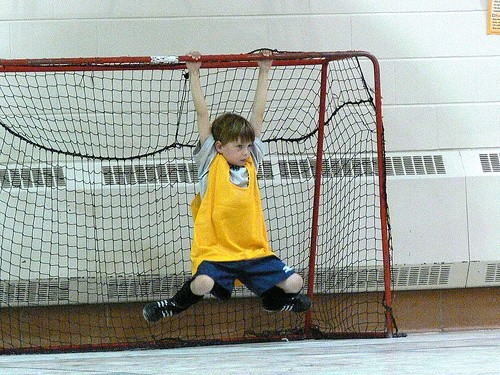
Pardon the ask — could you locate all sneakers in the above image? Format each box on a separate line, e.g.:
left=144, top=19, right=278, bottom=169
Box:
left=260, top=294, right=313, bottom=313
left=142, top=298, right=178, bottom=324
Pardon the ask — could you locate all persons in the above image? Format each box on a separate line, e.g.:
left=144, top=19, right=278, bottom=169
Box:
left=142, top=50, right=314, bottom=323
